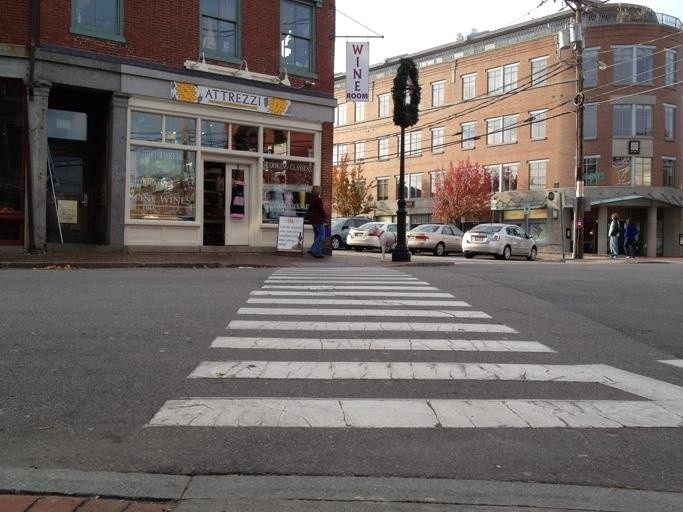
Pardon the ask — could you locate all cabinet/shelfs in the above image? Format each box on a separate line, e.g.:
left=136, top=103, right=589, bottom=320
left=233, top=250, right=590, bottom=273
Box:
left=204, top=178, right=224, bottom=235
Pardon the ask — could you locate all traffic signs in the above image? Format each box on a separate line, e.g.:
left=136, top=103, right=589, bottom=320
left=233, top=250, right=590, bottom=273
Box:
left=584, top=172, right=602, bottom=181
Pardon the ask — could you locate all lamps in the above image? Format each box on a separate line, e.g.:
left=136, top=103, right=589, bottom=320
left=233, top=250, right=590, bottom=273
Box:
left=194, top=50, right=292, bottom=87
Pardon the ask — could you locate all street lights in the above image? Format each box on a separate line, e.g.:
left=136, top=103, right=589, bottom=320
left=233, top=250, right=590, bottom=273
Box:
left=555, top=0, right=609, bottom=259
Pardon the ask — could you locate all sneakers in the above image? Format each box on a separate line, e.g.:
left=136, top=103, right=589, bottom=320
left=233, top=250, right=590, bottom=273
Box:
left=308, top=250, right=325, bottom=259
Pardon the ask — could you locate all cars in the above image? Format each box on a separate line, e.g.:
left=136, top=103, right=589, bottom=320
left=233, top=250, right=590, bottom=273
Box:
left=0, top=178, right=22, bottom=219
left=330, top=217, right=537, bottom=259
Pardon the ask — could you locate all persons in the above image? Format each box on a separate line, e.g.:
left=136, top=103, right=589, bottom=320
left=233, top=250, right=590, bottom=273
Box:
left=623, top=217, right=638, bottom=259
left=607, top=212, right=620, bottom=258
left=306, top=185, right=327, bottom=258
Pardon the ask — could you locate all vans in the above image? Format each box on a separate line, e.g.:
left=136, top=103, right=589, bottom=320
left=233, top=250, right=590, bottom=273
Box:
left=262, top=183, right=311, bottom=220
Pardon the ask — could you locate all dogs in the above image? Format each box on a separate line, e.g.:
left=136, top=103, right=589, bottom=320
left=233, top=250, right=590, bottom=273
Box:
left=368, top=225, right=413, bottom=261
